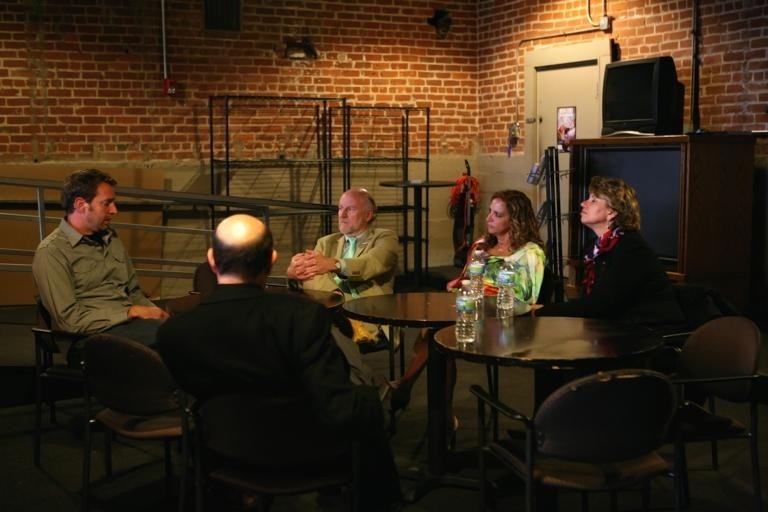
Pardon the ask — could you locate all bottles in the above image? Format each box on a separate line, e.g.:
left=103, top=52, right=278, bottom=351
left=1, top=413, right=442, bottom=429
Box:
left=469, top=249, right=486, bottom=304
left=495, top=256, right=516, bottom=309
left=455, top=280, right=476, bottom=345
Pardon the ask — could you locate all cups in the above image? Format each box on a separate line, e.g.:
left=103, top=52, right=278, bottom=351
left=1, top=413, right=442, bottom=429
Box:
left=526, top=163, right=544, bottom=185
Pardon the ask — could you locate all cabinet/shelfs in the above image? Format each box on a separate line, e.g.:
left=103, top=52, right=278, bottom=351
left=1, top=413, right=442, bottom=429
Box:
left=327, top=105, right=431, bottom=272
left=204, top=92, right=346, bottom=235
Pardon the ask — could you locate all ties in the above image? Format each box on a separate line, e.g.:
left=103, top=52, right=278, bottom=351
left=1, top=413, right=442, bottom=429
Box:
left=333, top=237, right=357, bottom=285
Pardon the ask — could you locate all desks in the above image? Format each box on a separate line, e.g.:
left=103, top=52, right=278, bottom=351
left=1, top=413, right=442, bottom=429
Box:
left=380, top=176, right=456, bottom=291
left=434, top=315, right=670, bottom=504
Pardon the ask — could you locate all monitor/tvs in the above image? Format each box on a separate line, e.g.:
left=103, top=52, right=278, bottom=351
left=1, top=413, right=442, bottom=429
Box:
left=602, top=56, right=685, bottom=135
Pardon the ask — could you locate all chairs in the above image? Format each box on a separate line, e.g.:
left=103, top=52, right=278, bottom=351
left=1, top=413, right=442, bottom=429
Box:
left=468, top=369, right=678, bottom=510
left=28, top=292, right=534, bottom=510
left=657, top=317, right=768, bottom=510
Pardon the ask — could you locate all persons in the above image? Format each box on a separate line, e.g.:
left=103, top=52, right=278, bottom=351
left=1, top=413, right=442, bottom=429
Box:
left=286, top=182, right=403, bottom=384
left=502, top=171, right=702, bottom=450
left=28, top=166, right=173, bottom=365
left=383, top=189, right=550, bottom=455
left=154, top=211, right=403, bottom=512
